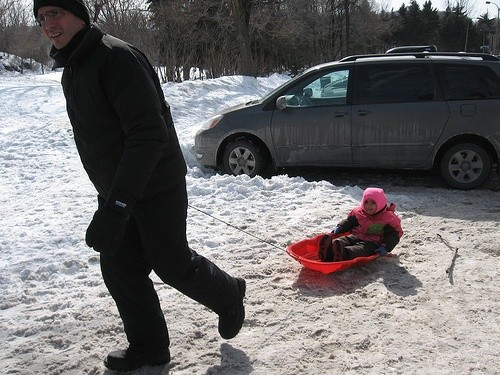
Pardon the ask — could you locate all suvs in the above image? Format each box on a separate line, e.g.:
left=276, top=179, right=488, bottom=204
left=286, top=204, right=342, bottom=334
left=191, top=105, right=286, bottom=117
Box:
left=194, top=46, right=500, bottom=190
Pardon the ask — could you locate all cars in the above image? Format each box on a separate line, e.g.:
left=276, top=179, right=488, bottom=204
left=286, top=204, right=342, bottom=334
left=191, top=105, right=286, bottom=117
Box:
left=321, top=74, right=348, bottom=99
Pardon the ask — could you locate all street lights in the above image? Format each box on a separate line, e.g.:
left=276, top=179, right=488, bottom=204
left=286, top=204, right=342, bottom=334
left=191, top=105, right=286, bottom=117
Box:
left=486, top=1, right=500, bottom=50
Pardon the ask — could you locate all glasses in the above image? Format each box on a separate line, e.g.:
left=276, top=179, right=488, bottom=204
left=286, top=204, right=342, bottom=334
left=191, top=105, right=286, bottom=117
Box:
left=35, top=9, right=68, bottom=23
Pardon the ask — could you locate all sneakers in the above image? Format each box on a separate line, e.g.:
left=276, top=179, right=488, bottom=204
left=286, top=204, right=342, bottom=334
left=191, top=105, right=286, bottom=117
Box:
left=104, top=347, right=170, bottom=372
left=218, top=278, right=246, bottom=340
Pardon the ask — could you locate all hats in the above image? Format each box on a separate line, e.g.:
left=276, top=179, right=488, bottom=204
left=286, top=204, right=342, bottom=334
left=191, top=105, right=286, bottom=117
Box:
left=361, top=188, right=387, bottom=215
left=33, top=0, right=90, bottom=27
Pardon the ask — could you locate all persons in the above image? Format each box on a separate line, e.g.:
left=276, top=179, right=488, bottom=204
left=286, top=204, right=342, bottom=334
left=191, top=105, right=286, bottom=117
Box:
left=33, top=1, right=246, bottom=372
left=318, top=188, right=403, bottom=264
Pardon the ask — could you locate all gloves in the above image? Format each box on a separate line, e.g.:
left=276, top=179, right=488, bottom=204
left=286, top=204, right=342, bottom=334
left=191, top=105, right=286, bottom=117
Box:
left=85, top=200, right=142, bottom=253
left=374, top=246, right=387, bottom=256
left=331, top=227, right=339, bottom=236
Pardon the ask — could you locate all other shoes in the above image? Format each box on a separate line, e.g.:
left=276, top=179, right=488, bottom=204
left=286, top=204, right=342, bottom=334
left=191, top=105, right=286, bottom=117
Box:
left=318, top=235, right=332, bottom=261
left=332, top=238, right=345, bottom=261
